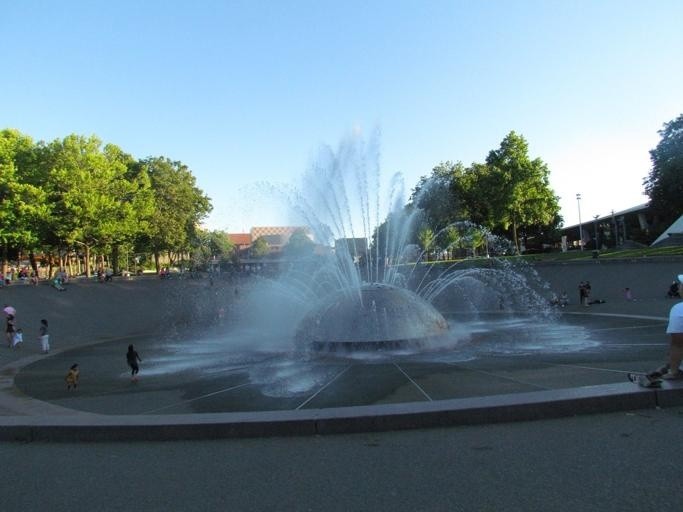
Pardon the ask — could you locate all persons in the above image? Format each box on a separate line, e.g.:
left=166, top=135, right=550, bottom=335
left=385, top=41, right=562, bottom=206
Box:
left=126, top=345, right=141, bottom=382
left=496, top=280, right=592, bottom=312
left=13, top=328, right=24, bottom=350
left=660, top=274, right=682, bottom=380
left=671, top=272, right=676, bottom=284
left=676, top=283, right=682, bottom=296
left=64, top=363, right=80, bottom=393
left=35, top=319, right=49, bottom=354
left=6, top=313, right=16, bottom=349
left=591, top=249, right=597, bottom=263
left=592, top=248, right=601, bottom=263
left=7, top=265, right=240, bottom=301
left=667, top=280, right=678, bottom=295
left=218, top=306, right=224, bottom=318
left=622, top=288, right=634, bottom=301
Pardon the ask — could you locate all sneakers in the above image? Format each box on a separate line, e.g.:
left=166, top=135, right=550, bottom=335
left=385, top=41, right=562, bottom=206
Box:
left=626, top=369, right=653, bottom=387
left=660, top=368, right=683, bottom=381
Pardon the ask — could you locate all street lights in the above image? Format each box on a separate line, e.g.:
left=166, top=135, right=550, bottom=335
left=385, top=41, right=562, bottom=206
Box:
left=60, top=239, right=92, bottom=278
left=593, top=214, right=600, bottom=250
left=576, top=192, right=584, bottom=251
left=125, top=245, right=130, bottom=271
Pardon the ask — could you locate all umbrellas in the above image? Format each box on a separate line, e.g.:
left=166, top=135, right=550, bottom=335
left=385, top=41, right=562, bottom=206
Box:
left=4, top=307, right=18, bottom=315
left=677, top=273, right=683, bottom=282
left=648, top=215, right=682, bottom=249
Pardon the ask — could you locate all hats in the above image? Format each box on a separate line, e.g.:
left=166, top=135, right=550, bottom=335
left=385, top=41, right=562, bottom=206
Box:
left=677, top=274, right=683, bottom=283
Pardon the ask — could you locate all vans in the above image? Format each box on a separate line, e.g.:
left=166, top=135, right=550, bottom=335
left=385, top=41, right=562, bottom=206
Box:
left=19, top=260, right=29, bottom=270
